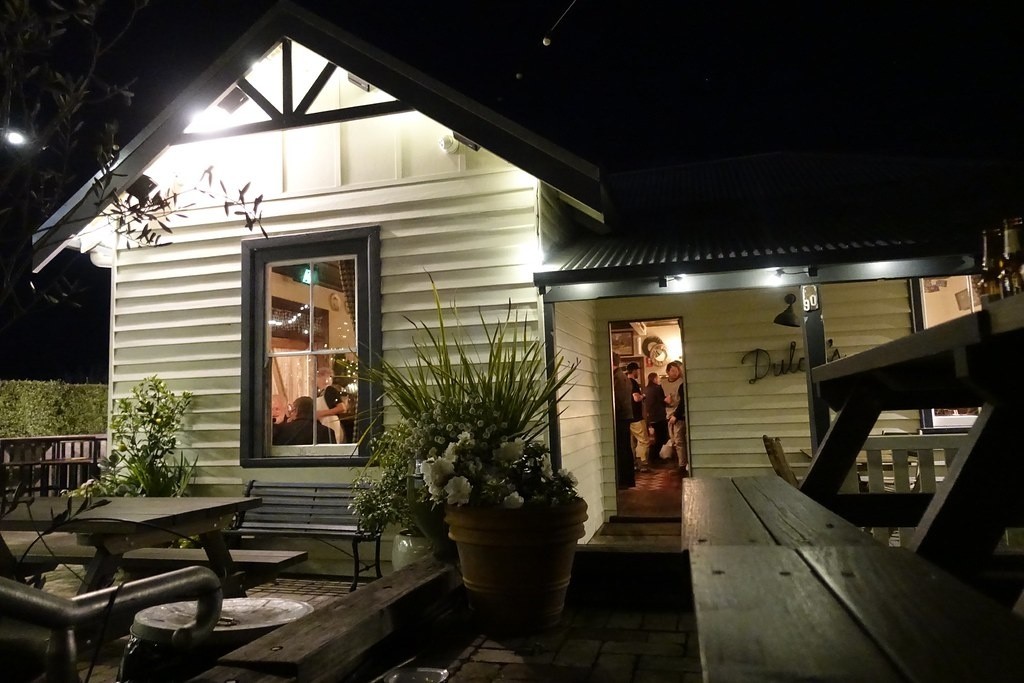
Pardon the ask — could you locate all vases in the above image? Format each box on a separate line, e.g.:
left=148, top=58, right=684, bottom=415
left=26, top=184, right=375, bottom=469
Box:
left=445, top=498, right=589, bottom=632
left=408, top=473, right=478, bottom=555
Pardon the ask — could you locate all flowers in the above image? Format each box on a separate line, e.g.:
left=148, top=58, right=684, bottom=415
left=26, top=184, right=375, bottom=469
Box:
left=425, top=435, right=578, bottom=510
left=320, top=265, right=582, bottom=466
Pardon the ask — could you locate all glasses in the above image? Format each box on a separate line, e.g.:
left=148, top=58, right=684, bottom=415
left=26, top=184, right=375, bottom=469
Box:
left=289, top=409, right=296, bottom=415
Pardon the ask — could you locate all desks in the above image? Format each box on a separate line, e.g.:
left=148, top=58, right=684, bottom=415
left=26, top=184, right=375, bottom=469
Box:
left=0, top=494, right=261, bottom=597
left=802, top=447, right=918, bottom=498
left=0, top=435, right=96, bottom=499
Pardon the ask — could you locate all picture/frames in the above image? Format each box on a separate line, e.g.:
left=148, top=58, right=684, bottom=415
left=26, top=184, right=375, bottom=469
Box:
left=611, top=331, right=635, bottom=356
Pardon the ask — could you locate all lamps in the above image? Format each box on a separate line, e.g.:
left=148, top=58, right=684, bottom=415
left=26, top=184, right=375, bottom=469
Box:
left=659, top=275, right=685, bottom=287
left=774, top=293, right=801, bottom=327
left=774, top=268, right=808, bottom=279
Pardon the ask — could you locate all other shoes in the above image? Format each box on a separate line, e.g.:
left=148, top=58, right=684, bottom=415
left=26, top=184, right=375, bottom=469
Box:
left=656, top=457, right=674, bottom=468
left=634, top=460, right=641, bottom=469
left=640, top=463, right=655, bottom=472
left=680, top=467, right=689, bottom=479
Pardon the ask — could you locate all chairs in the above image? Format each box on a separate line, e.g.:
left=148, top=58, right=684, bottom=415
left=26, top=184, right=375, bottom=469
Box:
left=761, top=435, right=804, bottom=490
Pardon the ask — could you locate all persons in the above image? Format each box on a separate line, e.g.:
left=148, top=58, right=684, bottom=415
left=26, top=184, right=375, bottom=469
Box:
left=273, top=397, right=336, bottom=445
left=675, top=361, right=682, bottom=371
left=627, top=363, right=651, bottom=472
left=317, top=367, right=347, bottom=443
left=660, top=362, right=687, bottom=475
left=642, top=372, right=669, bottom=443
left=613, top=352, right=635, bottom=491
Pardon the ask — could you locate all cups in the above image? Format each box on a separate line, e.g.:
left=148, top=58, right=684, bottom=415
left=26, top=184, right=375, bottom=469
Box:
left=980, top=218, right=1024, bottom=299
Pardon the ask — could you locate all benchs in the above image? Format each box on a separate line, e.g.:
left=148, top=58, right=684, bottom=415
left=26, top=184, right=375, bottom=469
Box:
left=680, top=299, right=1024, bottom=683
left=0, top=458, right=96, bottom=515
left=0, top=530, right=308, bottom=604
left=223, top=480, right=389, bottom=593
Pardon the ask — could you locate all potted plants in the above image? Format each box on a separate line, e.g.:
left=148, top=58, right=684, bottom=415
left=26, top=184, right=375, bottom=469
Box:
left=350, top=484, right=430, bottom=572
left=350, top=425, right=453, bottom=551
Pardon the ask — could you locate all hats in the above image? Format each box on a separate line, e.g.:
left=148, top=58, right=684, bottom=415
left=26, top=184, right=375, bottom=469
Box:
left=627, top=362, right=641, bottom=371
